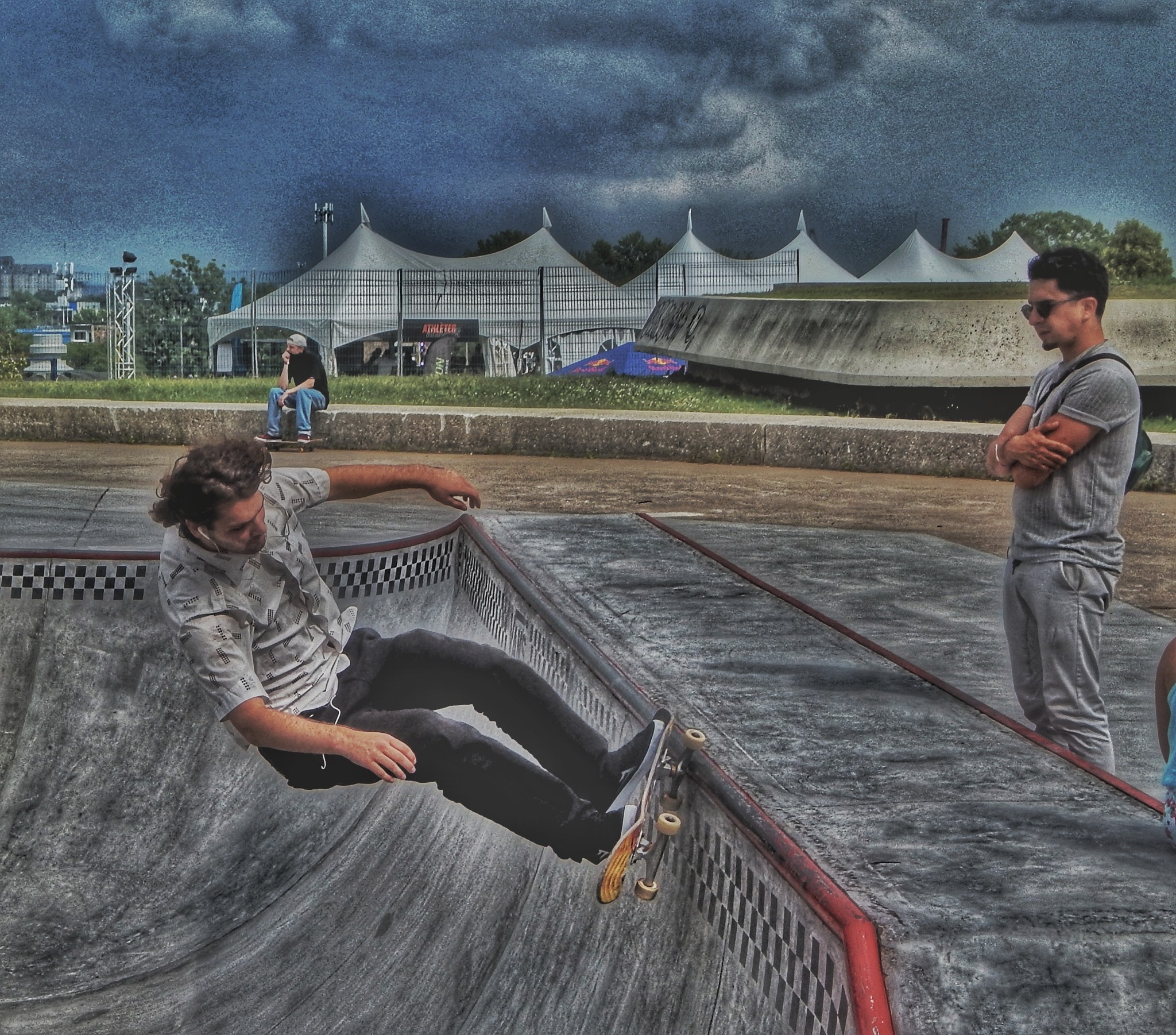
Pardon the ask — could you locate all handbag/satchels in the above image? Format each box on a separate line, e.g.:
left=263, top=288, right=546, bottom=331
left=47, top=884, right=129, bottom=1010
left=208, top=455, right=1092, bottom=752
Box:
left=1035, top=352, right=1154, bottom=499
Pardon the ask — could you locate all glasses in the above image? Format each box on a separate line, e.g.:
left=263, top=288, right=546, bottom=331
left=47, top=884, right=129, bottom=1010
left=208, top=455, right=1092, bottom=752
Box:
left=1021, top=294, right=1084, bottom=320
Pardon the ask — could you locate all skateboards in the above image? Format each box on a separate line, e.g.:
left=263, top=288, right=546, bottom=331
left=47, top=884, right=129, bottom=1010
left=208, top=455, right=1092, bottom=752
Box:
left=253, top=437, right=324, bottom=453
left=595, top=714, right=707, bottom=906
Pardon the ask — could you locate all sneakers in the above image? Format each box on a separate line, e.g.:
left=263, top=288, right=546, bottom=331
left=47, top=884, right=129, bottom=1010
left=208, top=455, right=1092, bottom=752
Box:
left=587, top=804, right=637, bottom=868
left=600, top=719, right=665, bottom=814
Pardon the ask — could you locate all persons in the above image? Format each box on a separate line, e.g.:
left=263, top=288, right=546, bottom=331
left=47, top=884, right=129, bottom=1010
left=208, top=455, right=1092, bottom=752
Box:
left=148, top=440, right=664, bottom=865
left=1155, top=636, right=1176, bottom=845
left=450, top=343, right=484, bottom=376
left=253, top=334, right=330, bottom=442
left=987, top=246, right=1138, bottom=779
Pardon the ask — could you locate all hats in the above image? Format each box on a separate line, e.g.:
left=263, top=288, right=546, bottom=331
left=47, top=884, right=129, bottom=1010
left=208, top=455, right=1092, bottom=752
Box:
left=286, top=334, right=308, bottom=348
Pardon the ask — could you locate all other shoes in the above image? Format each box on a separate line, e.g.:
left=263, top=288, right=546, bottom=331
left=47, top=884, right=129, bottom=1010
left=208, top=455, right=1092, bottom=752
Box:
left=255, top=434, right=282, bottom=442
left=297, top=434, right=311, bottom=443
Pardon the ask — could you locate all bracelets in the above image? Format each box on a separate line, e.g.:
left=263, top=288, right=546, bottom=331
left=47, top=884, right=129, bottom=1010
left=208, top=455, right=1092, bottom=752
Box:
left=995, top=443, right=1006, bottom=466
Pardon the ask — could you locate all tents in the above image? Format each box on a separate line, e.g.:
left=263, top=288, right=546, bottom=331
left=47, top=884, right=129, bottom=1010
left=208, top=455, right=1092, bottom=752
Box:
left=207, top=224, right=1040, bottom=379
